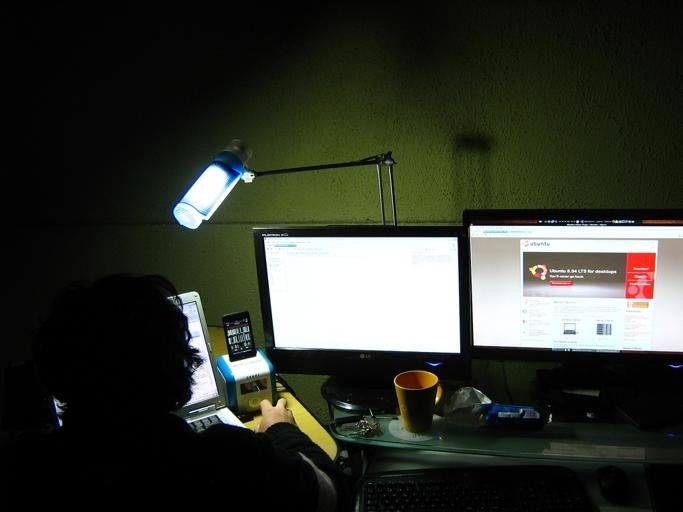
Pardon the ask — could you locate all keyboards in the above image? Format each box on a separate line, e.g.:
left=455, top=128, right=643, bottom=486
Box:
left=359, top=465, right=600, bottom=512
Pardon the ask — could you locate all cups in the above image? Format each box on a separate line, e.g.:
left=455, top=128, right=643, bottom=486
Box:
left=393, top=370, right=438, bottom=433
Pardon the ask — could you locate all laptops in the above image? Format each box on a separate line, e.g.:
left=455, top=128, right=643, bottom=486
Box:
left=52, top=291, right=247, bottom=433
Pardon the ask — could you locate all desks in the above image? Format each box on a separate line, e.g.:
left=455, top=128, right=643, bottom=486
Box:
left=325, top=370, right=683, bottom=470
left=203, top=319, right=340, bottom=479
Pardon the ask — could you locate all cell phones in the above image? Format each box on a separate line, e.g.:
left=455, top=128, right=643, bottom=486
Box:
left=222, top=310, right=256, bottom=362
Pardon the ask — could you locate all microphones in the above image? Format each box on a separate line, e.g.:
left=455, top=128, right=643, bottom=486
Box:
left=172, top=139, right=253, bottom=229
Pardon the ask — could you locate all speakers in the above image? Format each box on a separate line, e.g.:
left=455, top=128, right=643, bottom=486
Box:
left=657, top=355, right=682, bottom=390
left=421, top=353, right=455, bottom=380
left=217, top=349, right=278, bottom=414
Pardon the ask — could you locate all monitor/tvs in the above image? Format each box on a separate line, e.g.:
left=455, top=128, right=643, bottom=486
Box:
left=253, top=225, right=472, bottom=415
left=462, top=208, right=683, bottom=424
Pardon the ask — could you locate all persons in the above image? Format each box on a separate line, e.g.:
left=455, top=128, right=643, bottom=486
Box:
left=18, top=274, right=357, bottom=511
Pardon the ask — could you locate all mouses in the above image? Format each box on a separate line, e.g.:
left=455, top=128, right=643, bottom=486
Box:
left=597, top=465, right=636, bottom=506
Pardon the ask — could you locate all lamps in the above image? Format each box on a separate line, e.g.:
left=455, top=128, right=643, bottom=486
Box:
left=169, top=137, right=404, bottom=234
